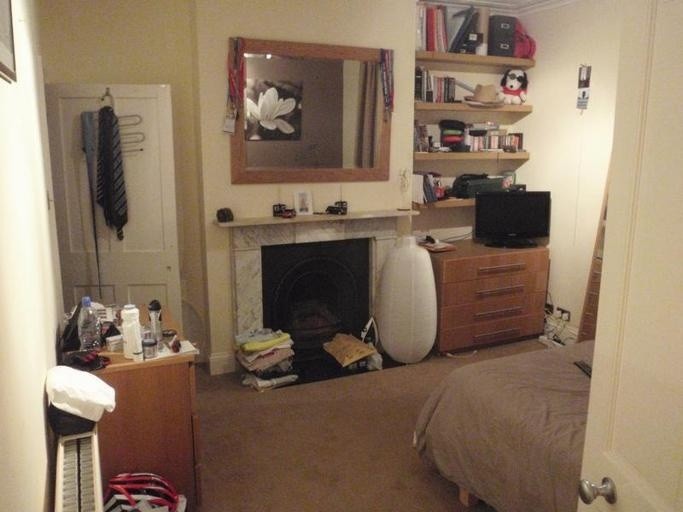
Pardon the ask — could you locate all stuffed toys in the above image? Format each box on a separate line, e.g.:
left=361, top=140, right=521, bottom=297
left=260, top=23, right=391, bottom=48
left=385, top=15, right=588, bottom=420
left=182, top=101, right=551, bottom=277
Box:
left=498, top=67, right=528, bottom=105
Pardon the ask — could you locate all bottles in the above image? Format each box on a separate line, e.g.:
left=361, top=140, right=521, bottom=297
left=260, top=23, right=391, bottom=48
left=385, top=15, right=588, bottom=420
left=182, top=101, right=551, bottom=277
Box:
left=76, top=294, right=102, bottom=350
left=142, top=337, right=156, bottom=359
left=119, top=304, right=143, bottom=360
left=162, top=329, right=178, bottom=344
left=434, top=181, right=444, bottom=199
left=146, top=298, right=163, bottom=350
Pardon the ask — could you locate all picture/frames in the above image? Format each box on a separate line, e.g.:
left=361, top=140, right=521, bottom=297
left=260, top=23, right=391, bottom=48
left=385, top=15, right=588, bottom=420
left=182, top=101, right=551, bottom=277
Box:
left=0, top=0, right=21, bottom=84
left=292, top=188, right=313, bottom=217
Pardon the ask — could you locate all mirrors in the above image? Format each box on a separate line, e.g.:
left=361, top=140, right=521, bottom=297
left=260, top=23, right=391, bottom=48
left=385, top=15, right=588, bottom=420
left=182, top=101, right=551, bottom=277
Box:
left=225, top=34, right=395, bottom=186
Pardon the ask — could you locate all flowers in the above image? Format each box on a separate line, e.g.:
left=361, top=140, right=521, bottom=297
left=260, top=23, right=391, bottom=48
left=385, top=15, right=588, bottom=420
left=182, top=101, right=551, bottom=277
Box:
left=244, top=81, right=300, bottom=140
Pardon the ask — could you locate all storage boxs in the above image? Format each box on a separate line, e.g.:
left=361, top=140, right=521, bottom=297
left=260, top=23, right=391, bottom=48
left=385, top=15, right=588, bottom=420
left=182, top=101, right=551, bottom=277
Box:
left=487, top=12, right=517, bottom=40
left=485, top=33, right=516, bottom=59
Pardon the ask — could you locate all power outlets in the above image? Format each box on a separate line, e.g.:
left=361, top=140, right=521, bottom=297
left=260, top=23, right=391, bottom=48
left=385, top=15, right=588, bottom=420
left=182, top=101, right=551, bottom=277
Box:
left=553, top=307, right=573, bottom=323
left=542, top=302, right=554, bottom=316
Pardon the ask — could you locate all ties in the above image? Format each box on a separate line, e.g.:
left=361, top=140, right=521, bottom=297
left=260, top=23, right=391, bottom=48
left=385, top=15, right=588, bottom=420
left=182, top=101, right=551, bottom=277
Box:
left=96, top=106, right=128, bottom=239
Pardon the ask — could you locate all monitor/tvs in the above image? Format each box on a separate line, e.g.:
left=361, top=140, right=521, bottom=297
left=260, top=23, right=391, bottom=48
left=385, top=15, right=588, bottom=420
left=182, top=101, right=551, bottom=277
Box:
left=475, top=188, right=552, bottom=248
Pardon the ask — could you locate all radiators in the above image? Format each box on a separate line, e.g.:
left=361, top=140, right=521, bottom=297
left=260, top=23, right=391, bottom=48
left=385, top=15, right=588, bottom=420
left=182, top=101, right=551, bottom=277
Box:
left=55, top=419, right=105, bottom=512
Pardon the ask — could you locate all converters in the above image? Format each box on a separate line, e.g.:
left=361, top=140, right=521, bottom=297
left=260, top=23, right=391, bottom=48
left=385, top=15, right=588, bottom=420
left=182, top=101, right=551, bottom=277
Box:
left=554, top=311, right=561, bottom=318
left=561, top=313, right=568, bottom=320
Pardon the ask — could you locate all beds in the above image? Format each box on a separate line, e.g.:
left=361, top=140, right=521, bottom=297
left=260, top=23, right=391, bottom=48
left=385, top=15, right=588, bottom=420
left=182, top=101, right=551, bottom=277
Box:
left=408, top=335, right=600, bottom=512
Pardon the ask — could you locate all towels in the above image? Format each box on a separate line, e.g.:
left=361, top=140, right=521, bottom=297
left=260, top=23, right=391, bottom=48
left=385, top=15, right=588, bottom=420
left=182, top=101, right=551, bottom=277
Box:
left=89, top=100, right=128, bottom=238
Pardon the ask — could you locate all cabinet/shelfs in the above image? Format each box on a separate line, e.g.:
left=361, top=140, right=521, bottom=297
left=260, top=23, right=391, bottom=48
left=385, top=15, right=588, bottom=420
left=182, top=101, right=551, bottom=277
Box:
left=426, top=235, right=551, bottom=355
left=52, top=301, right=209, bottom=511
left=411, top=48, right=536, bottom=214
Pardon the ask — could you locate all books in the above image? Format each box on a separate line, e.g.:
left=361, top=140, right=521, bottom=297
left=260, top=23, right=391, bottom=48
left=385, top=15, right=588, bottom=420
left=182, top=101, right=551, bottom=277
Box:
left=416, top=67, right=455, bottom=103
left=417, top=4, right=475, bottom=53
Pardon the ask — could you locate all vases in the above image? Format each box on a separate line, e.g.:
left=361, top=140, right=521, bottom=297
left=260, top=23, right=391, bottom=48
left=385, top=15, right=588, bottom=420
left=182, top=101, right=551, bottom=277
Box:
left=376, top=235, right=437, bottom=366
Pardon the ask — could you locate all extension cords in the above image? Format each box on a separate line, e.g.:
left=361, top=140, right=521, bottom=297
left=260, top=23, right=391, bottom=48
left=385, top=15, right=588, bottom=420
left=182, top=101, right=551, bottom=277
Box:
left=538, top=335, right=563, bottom=349
